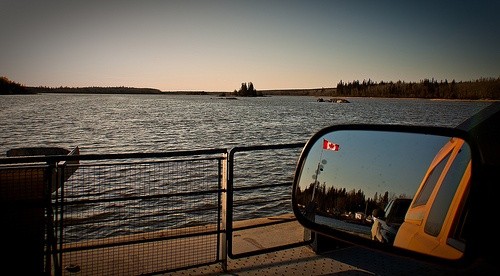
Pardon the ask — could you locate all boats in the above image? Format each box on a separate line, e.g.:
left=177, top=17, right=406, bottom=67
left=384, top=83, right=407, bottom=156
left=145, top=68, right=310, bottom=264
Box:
left=0, top=145, right=80, bottom=204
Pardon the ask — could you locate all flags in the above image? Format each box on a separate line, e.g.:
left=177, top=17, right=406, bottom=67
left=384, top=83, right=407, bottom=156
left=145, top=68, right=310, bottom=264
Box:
left=323, top=138, right=339, bottom=152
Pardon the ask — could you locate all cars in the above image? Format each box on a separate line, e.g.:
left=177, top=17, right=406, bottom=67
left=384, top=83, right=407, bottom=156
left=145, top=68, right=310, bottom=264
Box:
left=370, top=198, right=413, bottom=246
